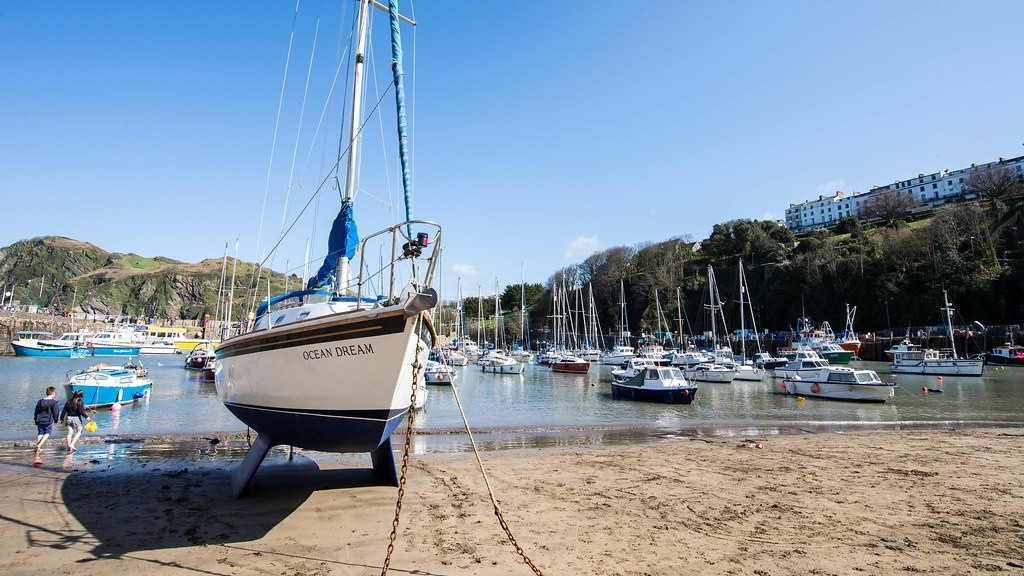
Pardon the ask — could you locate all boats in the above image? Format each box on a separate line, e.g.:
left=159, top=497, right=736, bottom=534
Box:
left=610, top=366, right=698, bottom=404
left=62, top=356, right=153, bottom=410
left=783, top=367, right=898, bottom=401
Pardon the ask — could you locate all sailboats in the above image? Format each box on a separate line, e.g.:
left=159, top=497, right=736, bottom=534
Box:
left=0, top=258, right=1024, bottom=386
left=214, top=0, right=442, bottom=499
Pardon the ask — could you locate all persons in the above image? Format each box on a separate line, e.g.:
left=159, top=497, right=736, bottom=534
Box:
left=60, top=389, right=90, bottom=451
left=34, top=387, right=59, bottom=453
